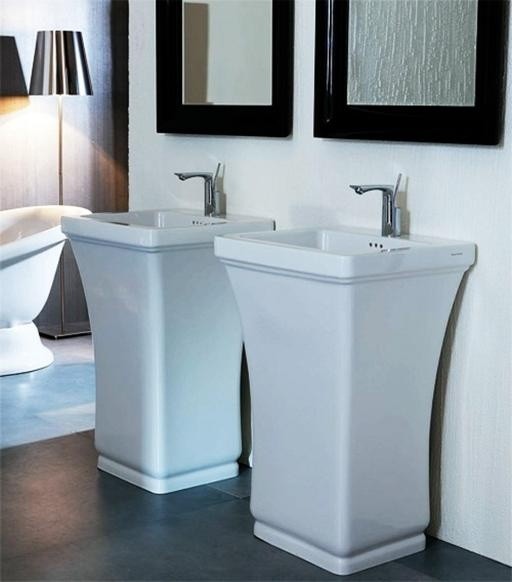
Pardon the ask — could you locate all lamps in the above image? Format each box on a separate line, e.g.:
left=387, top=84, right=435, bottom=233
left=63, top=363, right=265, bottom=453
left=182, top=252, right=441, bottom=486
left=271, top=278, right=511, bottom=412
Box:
left=1, top=35, right=30, bottom=120
left=23, top=27, right=99, bottom=341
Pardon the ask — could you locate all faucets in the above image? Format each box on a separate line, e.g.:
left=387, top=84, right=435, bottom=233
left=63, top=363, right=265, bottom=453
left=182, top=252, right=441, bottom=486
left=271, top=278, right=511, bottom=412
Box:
left=350, top=173, right=404, bottom=236
left=173, top=163, right=222, bottom=216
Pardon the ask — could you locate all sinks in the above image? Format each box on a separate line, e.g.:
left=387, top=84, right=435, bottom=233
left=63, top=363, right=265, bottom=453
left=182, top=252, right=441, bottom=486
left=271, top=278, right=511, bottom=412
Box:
left=213, top=225, right=477, bottom=279
left=61, top=209, right=275, bottom=248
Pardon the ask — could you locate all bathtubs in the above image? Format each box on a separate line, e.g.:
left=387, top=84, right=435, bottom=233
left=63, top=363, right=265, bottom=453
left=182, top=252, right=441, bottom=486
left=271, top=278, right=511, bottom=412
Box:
left=0, top=205, right=93, bottom=376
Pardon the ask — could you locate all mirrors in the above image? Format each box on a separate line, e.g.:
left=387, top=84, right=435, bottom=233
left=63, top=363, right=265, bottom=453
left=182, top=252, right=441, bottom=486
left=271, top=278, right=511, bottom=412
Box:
left=311, top=0, right=510, bottom=149
left=154, top=1, right=296, bottom=139
